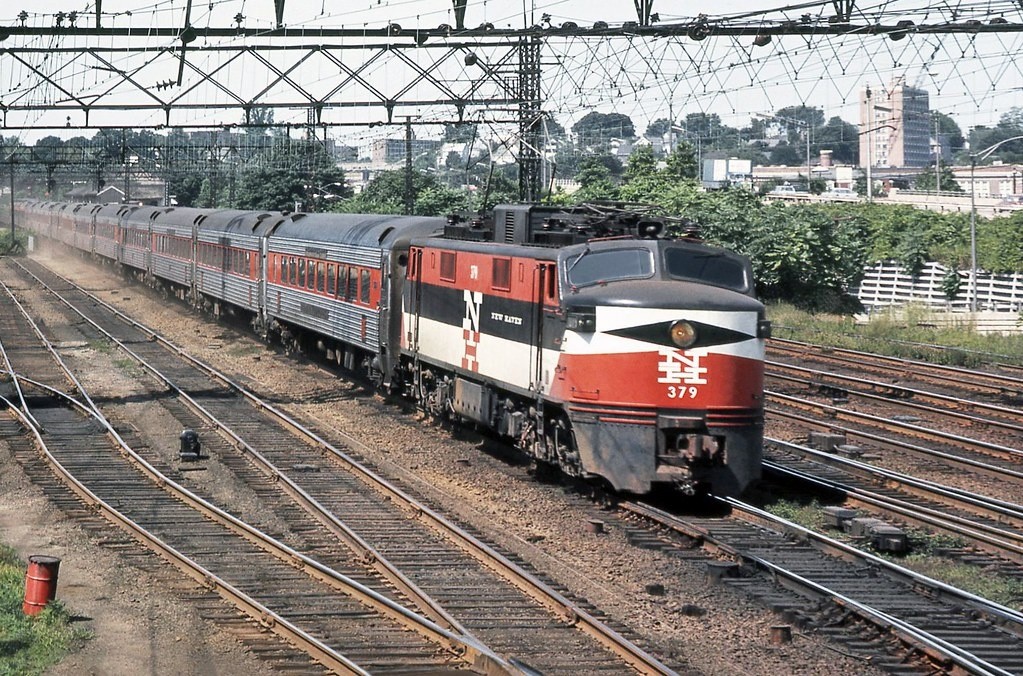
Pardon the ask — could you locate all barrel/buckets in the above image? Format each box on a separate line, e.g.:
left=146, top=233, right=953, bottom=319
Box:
left=22, top=555, right=61, bottom=617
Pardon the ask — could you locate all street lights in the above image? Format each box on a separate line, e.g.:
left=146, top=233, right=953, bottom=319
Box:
left=669, top=126, right=702, bottom=181
left=757, top=113, right=810, bottom=191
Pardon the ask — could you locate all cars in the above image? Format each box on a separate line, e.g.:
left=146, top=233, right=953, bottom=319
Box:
left=830, top=187, right=859, bottom=198
left=778, top=186, right=795, bottom=194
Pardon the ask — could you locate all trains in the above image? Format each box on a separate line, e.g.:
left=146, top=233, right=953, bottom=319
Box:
left=0, top=197, right=774, bottom=497
